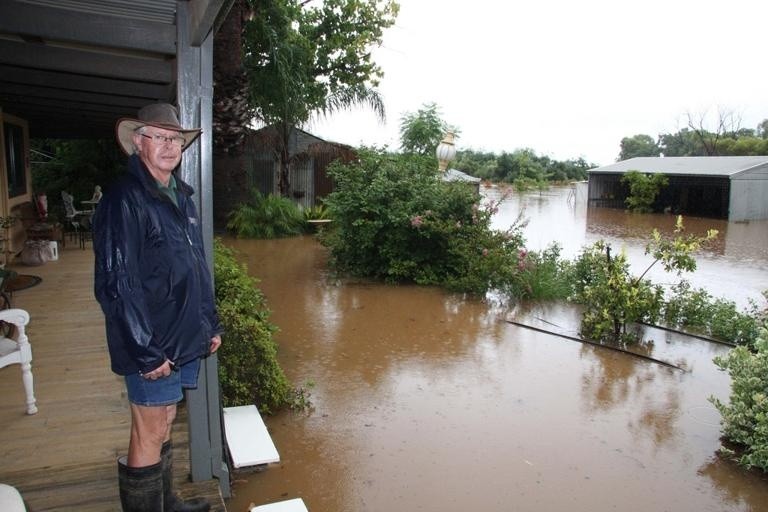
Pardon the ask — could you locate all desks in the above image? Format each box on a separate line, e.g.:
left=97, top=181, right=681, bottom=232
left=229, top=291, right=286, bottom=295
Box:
left=306, top=219, right=334, bottom=236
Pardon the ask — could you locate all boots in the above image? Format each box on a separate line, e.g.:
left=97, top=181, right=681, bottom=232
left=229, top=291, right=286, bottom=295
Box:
left=117, top=455, right=163, bottom=512
left=161, top=439, right=210, bottom=512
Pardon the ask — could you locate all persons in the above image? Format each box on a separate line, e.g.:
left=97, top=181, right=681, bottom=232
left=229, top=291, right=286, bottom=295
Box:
left=91, top=103, right=222, bottom=511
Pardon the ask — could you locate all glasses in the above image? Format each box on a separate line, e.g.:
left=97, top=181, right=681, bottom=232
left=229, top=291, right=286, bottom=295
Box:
left=141, top=133, right=186, bottom=147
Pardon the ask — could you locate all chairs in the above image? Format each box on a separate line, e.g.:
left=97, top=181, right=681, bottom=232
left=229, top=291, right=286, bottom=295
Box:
left=0, top=187, right=104, bottom=415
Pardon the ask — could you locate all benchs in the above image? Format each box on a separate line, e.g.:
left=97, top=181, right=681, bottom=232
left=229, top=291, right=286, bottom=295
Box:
left=250, top=497, right=310, bottom=512
left=220, top=403, right=281, bottom=474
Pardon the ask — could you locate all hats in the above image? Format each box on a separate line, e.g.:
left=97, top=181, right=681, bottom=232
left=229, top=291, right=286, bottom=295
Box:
left=115, top=103, right=202, bottom=159
